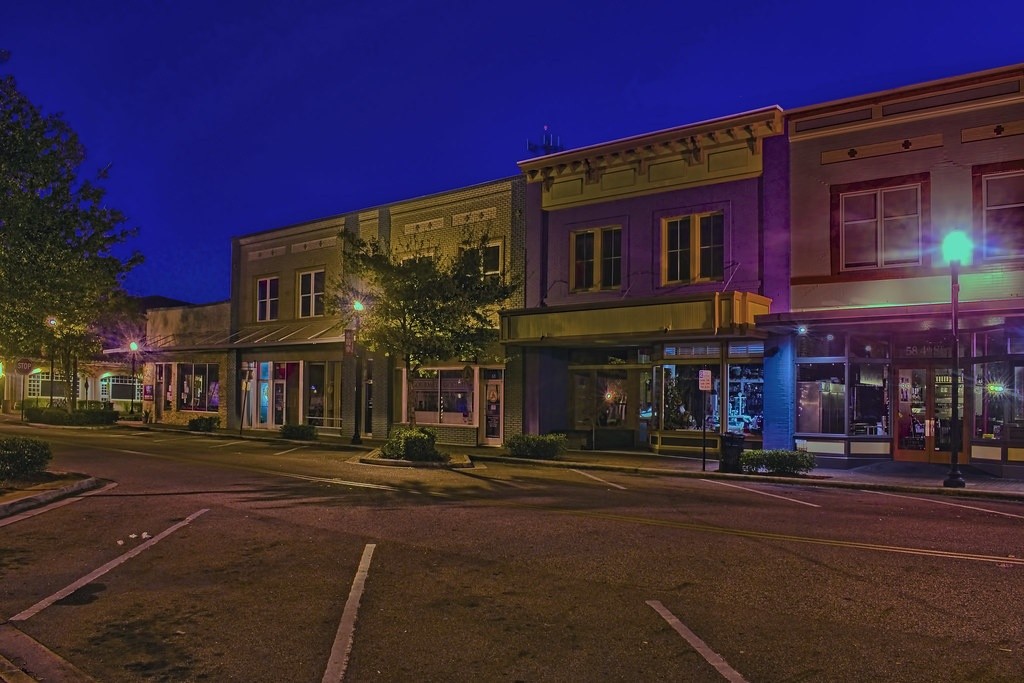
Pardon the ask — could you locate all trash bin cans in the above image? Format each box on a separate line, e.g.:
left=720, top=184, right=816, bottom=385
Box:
left=719, top=431, right=746, bottom=472
left=2, top=400, right=11, bottom=413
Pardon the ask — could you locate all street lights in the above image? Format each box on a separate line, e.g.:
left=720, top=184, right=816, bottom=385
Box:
left=349, top=302, right=365, bottom=444
left=942, top=231, right=973, bottom=487
left=128, top=341, right=138, bottom=414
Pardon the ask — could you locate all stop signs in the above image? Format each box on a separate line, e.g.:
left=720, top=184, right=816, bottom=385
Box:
left=15, top=359, right=33, bottom=375
